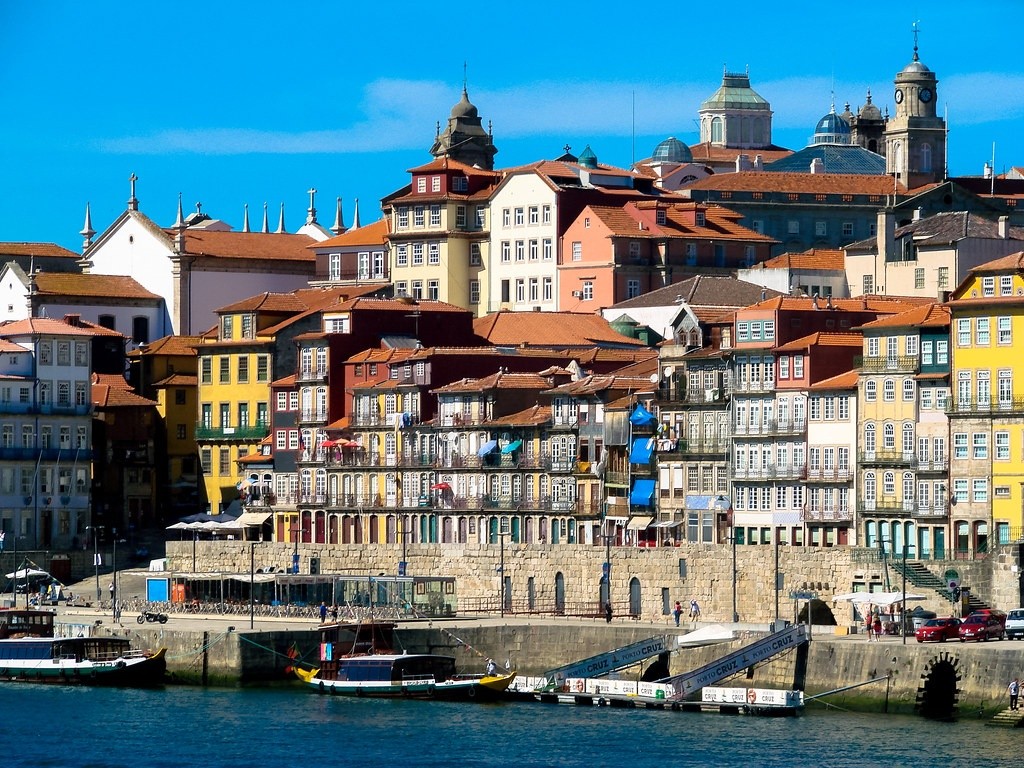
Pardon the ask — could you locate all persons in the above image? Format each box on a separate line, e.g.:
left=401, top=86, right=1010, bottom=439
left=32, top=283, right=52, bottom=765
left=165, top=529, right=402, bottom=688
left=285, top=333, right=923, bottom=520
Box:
left=486, top=657, right=497, bottom=677
left=29, top=590, right=73, bottom=606
left=604, top=599, right=613, bottom=624
left=873, top=613, right=881, bottom=641
left=1008, top=678, right=1019, bottom=710
left=108, top=582, right=121, bottom=623
left=672, top=599, right=700, bottom=627
left=319, top=601, right=338, bottom=623
left=865, top=613, right=872, bottom=642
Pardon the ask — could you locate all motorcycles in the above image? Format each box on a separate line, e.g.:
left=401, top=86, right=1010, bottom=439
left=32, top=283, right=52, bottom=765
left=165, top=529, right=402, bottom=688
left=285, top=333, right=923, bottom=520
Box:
left=137, top=609, right=169, bottom=624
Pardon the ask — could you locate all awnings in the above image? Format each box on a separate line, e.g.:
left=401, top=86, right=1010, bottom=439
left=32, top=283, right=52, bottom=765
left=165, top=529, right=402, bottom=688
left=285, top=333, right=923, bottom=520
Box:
left=629, top=438, right=654, bottom=463
left=649, top=520, right=685, bottom=528
left=237, top=477, right=269, bottom=495
left=630, top=480, right=655, bottom=505
left=477, top=439, right=521, bottom=457
left=626, top=516, right=654, bottom=530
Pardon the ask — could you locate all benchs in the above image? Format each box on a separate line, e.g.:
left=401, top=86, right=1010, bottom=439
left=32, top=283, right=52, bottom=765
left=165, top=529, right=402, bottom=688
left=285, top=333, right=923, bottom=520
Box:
left=25, top=598, right=58, bottom=605
left=61, top=601, right=95, bottom=607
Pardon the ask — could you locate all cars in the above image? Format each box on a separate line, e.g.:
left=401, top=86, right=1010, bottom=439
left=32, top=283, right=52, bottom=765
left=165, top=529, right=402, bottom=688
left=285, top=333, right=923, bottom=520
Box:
left=969, top=609, right=1007, bottom=629
left=916, top=618, right=963, bottom=643
left=959, top=614, right=1004, bottom=643
left=1005, top=609, right=1024, bottom=640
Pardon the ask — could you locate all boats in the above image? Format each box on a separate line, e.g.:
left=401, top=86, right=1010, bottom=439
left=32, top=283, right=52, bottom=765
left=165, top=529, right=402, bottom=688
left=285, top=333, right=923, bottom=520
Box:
left=287, top=570, right=517, bottom=699
left=0, top=555, right=168, bottom=686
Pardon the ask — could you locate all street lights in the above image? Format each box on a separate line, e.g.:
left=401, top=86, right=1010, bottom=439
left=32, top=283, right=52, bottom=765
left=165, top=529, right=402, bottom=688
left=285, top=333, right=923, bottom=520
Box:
left=86, top=526, right=106, bottom=600
left=595, top=535, right=610, bottom=602
left=14, top=537, right=25, bottom=606
left=902, top=545, right=916, bottom=644
left=491, top=533, right=503, bottom=618
left=722, top=537, right=737, bottom=622
left=113, top=539, right=127, bottom=624
left=250, top=542, right=262, bottom=629
left=393, top=531, right=406, bottom=601
left=717, top=494, right=733, bottom=545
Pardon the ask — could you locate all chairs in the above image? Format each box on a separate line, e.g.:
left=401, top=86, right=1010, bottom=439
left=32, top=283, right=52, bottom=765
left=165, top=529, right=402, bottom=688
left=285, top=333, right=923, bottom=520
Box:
left=97, top=599, right=407, bottom=619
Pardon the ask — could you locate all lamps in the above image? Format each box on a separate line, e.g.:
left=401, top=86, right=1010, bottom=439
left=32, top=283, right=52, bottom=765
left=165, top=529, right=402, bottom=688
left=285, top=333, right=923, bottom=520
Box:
left=940, top=485, right=952, bottom=493
left=986, top=475, right=990, bottom=481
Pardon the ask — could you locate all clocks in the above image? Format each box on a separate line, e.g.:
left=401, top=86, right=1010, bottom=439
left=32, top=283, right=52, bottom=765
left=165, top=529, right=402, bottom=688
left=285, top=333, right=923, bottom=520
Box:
left=919, top=88, right=933, bottom=104
left=894, top=89, right=903, bottom=104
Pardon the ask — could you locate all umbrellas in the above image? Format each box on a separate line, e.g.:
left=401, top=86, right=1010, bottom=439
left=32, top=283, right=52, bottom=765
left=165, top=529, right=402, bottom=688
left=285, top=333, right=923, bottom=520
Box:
left=319, top=437, right=359, bottom=465
left=165, top=513, right=251, bottom=541
left=832, top=590, right=927, bottom=622
left=4, top=568, right=51, bottom=593
left=429, top=482, right=450, bottom=500
